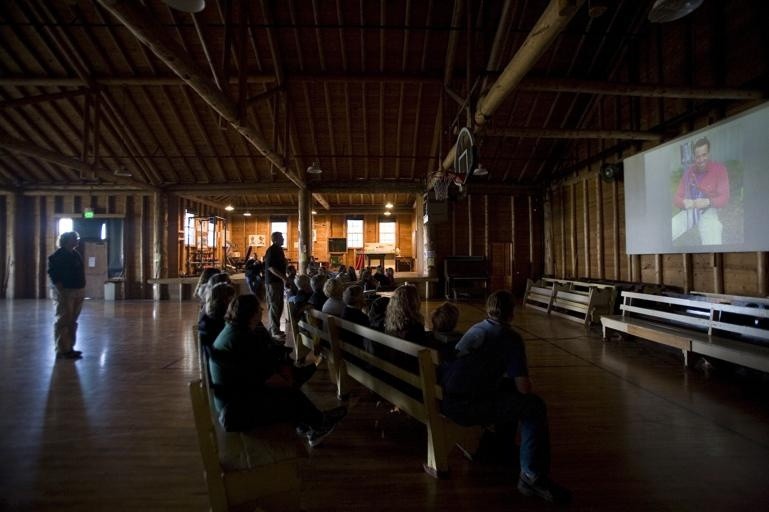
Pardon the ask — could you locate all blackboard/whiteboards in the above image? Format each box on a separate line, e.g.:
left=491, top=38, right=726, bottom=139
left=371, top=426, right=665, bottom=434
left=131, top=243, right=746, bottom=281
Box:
left=327, top=237, right=348, bottom=254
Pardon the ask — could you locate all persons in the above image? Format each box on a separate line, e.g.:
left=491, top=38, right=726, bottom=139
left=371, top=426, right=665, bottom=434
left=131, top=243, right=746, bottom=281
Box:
left=672, top=138, right=730, bottom=245
left=48, top=231, right=85, bottom=358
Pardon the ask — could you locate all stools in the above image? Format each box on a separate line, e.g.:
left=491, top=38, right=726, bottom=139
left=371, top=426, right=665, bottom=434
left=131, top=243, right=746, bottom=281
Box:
left=327, top=251, right=348, bottom=265
left=395, top=256, right=413, bottom=273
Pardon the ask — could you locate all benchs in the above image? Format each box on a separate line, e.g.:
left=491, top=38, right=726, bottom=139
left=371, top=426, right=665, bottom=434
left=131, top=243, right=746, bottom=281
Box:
left=284, top=300, right=456, bottom=512
left=523, top=277, right=768, bottom=374
left=187, top=324, right=312, bottom=511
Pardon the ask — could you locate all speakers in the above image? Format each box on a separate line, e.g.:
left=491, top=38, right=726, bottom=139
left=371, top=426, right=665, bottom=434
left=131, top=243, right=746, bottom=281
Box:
left=600, top=163, right=623, bottom=182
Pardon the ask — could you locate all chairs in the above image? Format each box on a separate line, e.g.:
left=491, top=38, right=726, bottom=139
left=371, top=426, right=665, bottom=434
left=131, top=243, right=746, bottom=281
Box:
left=233, top=246, right=252, bottom=273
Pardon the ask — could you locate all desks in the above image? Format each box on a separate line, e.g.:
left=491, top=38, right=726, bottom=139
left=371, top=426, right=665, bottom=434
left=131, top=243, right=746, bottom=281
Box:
left=356, top=253, right=399, bottom=271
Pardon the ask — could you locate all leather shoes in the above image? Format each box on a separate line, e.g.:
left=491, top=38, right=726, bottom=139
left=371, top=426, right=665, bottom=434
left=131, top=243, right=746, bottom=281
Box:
left=56, top=350, right=83, bottom=361
left=517, top=472, right=574, bottom=506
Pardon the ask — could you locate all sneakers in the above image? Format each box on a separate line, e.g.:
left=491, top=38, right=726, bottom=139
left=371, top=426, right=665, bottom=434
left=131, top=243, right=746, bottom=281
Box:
left=304, top=424, right=337, bottom=450
left=323, top=405, right=350, bottom=424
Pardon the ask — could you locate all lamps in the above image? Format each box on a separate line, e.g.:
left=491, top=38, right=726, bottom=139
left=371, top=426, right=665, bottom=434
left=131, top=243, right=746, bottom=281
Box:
left=383, top=211, right=391, bottom=217
left=242, top=209, right=252, bottom=217
left=311, top=209, right=318, bottom=215
left=472, top=152, right=489, bottom=176
left=384, top=201, right=394, bottom=208
left=113, top=164, right=133, bottom=177
left=306, top=157, right=323, bottom=174
left=224, top=204, right=235, bottom=212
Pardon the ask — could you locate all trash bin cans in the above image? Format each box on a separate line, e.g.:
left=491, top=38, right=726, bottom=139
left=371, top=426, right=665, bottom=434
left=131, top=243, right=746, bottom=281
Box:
left=103, top=280, right=122, bottom=301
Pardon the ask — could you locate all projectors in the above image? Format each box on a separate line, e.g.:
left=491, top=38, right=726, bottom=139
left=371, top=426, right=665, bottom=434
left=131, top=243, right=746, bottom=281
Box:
left=427, top=191, right=464, bottom=203
